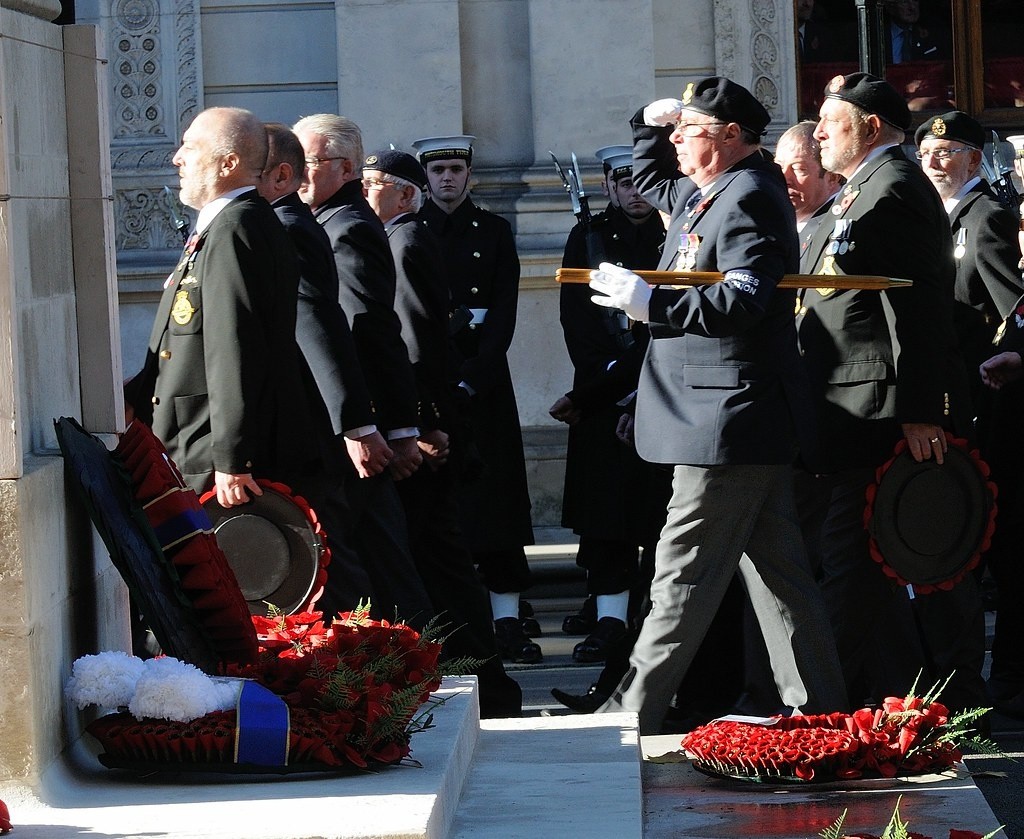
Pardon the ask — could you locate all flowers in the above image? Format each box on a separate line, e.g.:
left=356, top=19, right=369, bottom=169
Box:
left=61, top=648, right=355, bottom=764
left=852, top=658, right=1017, bottom=780
left=681, top=709, right=861, bottom=786
left=250, top=579, right=494, bottom=772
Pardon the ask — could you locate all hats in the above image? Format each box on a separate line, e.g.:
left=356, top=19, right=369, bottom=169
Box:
left=915, top=111, right=986, bottom=151
left=596, top=145, right=638, bottom=178
left=825, top=73, right=912, bottom=132
left=363, top=150, right=429, bottom=192
left=411, top=134, right=477, bottom=160
left=682, top=77, right=772, bottom=137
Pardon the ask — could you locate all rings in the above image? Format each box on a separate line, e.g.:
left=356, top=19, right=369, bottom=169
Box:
left=931, top=437, right=939, bottom=443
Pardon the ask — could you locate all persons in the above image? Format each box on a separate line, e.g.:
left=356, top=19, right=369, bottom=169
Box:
left=141, top=107, right=542, bottom=720
left=552, top=77, right=1024, bottom=746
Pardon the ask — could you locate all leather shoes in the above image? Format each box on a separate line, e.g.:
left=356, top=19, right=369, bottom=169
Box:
left=491, top=593, right=628, bottom=713
left=662, top=674, right=746, bottom=736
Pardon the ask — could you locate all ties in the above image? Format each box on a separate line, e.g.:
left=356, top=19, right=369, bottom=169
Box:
left=178, top=227, right=200, bottom=265
left=687, top=194, right=705, bottom=211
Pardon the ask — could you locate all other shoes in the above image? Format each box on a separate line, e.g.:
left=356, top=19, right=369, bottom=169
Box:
left=982, top=673, right=1024, bottom=699
left=998, top=694, right=1024, bottom=721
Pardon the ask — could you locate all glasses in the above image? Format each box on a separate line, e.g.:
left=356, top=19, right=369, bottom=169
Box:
left=299, top=156, right=348, bottom=169
left=915, top=148, right=970, bottom=160
left=675, top=121, right=728, bottom=132
left=363, top=178, right=403, bottom=189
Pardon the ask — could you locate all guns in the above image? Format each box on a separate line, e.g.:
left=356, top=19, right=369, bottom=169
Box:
left=982, top=130, right=1021, bottom=215
left=547, top=148, right=640, bottom=354
left=162, top=183, right=191, bottom=241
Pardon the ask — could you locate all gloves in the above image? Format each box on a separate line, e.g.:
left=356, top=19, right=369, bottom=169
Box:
left=589, top=262, right=651, bottom=324
